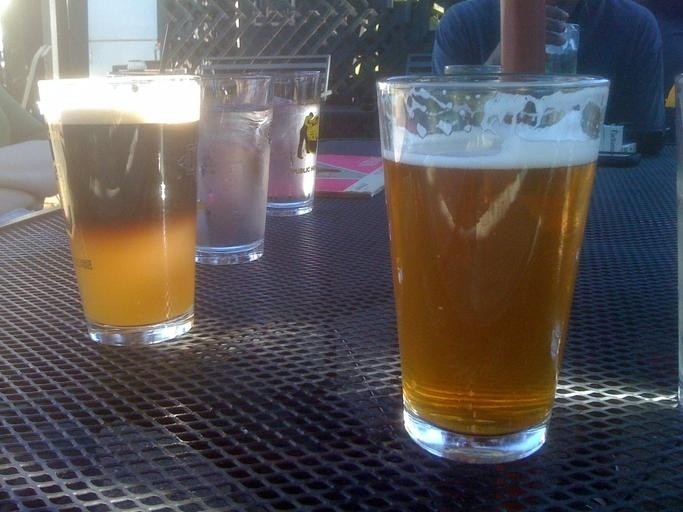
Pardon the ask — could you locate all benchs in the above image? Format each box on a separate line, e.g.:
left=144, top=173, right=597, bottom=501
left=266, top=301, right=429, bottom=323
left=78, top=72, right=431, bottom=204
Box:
left=197, top=51, right=334, bottom=110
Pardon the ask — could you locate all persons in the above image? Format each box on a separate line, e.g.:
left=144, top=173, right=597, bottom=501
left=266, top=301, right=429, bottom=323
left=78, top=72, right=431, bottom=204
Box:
left=0, top=83, right=62, bottom=230
left=296, top=112, right=315, bottom=160
left=430, top=0, right=669, bottom=161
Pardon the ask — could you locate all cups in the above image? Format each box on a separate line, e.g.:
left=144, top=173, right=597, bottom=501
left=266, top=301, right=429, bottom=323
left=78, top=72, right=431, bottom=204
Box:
left=376, top=73, right=610, bottom=464
left=35, top=74, right=196, bottom=349
left=545, top=23, right=581, bottom=55
left=128, top=60, right=147, bottom=71
left=189, top=77, right=274, bottom=267
left=237, top=73, right=323, bottom=217
left=443, top=63, right=503, bottom=75
